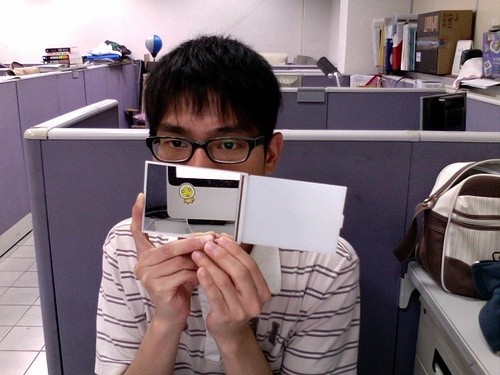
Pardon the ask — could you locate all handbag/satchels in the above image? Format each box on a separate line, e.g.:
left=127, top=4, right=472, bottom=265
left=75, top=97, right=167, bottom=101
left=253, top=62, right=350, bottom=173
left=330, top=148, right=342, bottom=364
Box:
left=392, top=157, right=500, bottom=296
left=470, top=253, right=500, bottom=356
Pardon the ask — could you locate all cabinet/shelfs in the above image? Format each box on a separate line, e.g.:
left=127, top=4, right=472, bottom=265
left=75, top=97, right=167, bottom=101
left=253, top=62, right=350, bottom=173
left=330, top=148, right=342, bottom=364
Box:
left=416, top=9, right=472, bottom=75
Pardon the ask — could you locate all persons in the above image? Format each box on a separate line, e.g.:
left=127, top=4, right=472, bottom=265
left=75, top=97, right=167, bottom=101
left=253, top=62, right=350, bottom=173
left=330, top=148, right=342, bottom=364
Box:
left=93, top=35, right=363, bottom=375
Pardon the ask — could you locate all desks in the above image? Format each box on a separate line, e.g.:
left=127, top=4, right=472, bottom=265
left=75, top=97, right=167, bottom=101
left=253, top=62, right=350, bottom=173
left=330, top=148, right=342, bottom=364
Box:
left=407, top=260, right=500, bottom=375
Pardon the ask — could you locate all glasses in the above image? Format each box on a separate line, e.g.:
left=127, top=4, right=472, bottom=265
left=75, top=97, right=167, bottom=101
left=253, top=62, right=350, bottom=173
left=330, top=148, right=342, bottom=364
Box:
left=145, top=135, right=266, bottom=164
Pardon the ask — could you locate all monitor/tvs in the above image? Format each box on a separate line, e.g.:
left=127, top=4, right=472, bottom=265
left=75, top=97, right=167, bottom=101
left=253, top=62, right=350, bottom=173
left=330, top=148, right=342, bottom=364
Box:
left=419, top=91, right=467, bottom=131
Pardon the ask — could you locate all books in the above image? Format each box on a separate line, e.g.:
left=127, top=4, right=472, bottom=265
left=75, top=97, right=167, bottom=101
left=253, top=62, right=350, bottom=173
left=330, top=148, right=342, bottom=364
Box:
left=42, top=46, right=85, bottom=67
left=392, top=23, right=416, bottom=71
